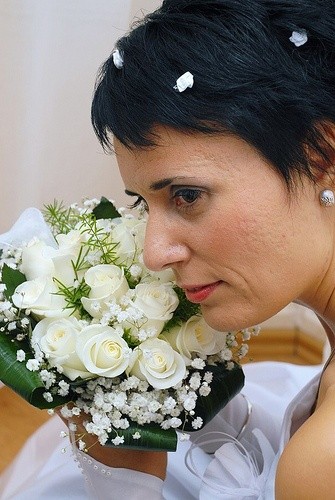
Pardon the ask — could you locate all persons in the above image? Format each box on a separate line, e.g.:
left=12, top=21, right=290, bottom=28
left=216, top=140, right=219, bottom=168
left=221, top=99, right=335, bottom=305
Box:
left=0, top=0, right=335, bottom=500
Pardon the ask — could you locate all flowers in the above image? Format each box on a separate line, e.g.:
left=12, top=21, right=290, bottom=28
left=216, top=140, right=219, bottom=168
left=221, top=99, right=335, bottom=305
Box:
left=0, top=197, right=262, bottom=453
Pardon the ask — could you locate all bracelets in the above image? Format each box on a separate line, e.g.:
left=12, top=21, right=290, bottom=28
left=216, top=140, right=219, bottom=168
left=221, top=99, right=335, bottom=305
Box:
left=205, top=393, right=252, bottom=456
